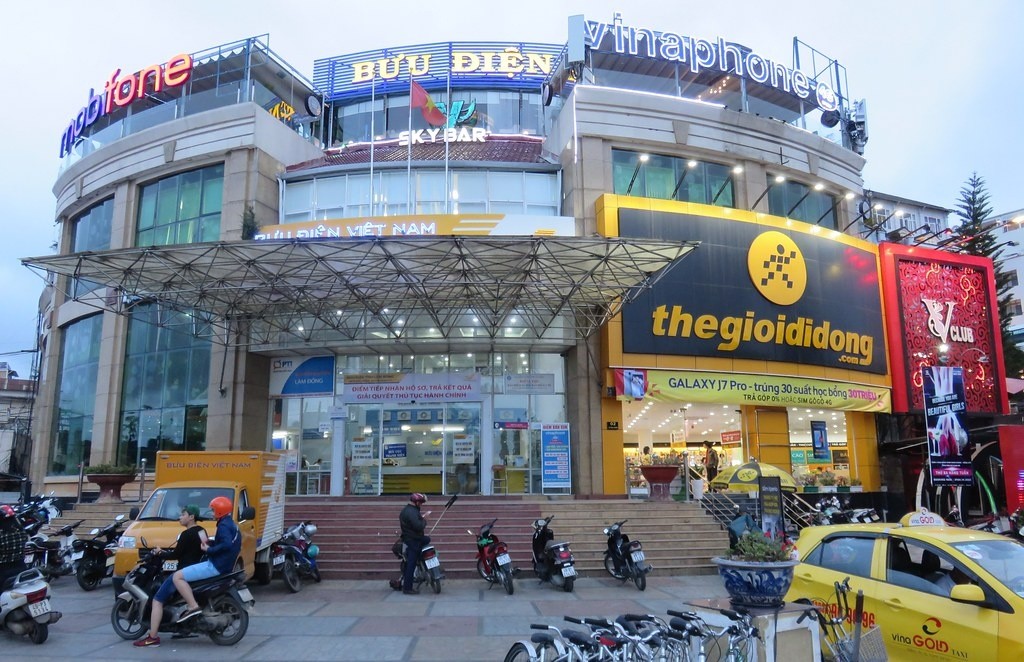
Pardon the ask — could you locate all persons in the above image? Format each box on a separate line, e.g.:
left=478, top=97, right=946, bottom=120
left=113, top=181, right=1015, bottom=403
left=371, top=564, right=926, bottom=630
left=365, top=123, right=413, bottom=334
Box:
left=133, top=504, right=208, bottom=649
left=643, top=446, right=653, bottom=466
left=398, top=492, right=432, bottom=595
left=455, top=462, right=471, bottom=495
left=473, top=448, right=481, bottom=493
left=701, top=457, right=707, bottom=492
left=312, top=458, right=323, bottom=467
left=172, top=497, right=243, bottom=622
left=929, top=366, right=961, bottom=457
left=702, top=440, right=719, bottom=493
left=301, top=455, right=311, bottom=467
left=0, top=504, right=29, bottom=588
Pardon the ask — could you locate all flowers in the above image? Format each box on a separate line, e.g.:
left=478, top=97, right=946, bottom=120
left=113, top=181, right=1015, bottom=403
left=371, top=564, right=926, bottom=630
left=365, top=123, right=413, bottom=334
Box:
left=800, top=473, right=862, bottom=487
left=723, top=530, right=800, bottom=563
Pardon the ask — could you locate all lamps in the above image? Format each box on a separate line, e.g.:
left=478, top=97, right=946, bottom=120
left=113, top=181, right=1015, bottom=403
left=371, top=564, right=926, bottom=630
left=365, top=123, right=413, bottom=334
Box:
left=622, top=151, right=1024, bottom=264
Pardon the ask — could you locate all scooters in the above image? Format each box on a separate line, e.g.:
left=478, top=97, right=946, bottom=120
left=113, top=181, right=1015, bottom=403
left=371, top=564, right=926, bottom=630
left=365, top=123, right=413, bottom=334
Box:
left=0, top=569, right=63, bottom=643
left=24, top=519, right=85, bottom=583
left=603, top=519, right=653, bottom=590
left=529, top=515, right=578, bottom=591
left=72, top=515, right=130, bottom=591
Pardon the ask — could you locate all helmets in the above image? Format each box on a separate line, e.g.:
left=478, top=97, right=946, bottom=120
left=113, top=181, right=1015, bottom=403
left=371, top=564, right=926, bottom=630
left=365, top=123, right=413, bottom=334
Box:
left=0, top=506, right=17, bottom=523
left=210, top=497, right=232, bottom=518
left=411, top=493, right=427, bottom=503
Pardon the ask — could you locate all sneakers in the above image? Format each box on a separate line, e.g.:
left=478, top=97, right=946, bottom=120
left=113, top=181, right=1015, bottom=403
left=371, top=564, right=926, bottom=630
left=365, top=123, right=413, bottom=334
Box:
left=133, top=635, right=161, bottom=648
left=176, top=609, right=202, bottom=623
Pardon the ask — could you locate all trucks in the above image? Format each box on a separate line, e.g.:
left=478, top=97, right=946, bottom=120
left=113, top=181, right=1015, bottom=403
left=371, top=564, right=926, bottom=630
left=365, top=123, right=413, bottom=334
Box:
left=114, top=450, right=289, bottom=604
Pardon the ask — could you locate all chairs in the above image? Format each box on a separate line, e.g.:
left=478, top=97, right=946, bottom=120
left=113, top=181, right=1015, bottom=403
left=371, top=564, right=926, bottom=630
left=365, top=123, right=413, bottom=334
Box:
left=490, top=464, right=510, bottom=498
left=891, top=546, right=957, bottom=598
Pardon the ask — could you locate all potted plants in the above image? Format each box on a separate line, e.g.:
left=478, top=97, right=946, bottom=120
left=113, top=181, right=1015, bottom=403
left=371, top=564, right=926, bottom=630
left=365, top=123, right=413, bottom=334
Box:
left=638, top=452, right=681, bottom=503
left=86, top=464, right=137, bottom=505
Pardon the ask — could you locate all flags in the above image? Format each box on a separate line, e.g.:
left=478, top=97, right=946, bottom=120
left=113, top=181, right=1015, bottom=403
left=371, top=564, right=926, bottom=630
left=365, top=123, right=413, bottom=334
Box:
left=411, top=79, right=446, bottom=128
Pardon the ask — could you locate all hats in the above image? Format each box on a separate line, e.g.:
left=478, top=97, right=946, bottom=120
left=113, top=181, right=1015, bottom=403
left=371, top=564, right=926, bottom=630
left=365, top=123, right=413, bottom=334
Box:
left=182, top=504, right=204, bottom=522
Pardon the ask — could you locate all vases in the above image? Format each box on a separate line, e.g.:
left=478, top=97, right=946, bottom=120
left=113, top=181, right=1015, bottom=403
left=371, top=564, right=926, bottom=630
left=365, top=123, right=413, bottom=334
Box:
left=802, top=485, right=863, bottom=493
left=710, top=555, right=801, bottom=611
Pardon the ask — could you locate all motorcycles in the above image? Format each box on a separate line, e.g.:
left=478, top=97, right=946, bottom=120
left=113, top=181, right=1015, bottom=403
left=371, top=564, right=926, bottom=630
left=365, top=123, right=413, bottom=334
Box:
left=466, top=518, right=521, bottom=596
left=11, top=491, right=63, bottom=534
left=272, top=514, right=322, bottom=593
left=111, top=534, right=254, bottom=646
left=393, top=528, right=443, bottom=594
left=786, top=494, right=881, bottom=530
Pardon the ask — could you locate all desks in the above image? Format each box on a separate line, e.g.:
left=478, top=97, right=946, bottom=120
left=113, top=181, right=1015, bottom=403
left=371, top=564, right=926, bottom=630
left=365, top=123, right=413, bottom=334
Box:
left=287, top=468, right=332, bottom=495
left=498, top=463, right=530, bottom=493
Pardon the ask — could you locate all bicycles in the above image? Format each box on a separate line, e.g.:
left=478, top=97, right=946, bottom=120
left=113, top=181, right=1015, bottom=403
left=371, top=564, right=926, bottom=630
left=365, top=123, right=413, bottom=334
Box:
left=504, top=574, right=889, bottom=662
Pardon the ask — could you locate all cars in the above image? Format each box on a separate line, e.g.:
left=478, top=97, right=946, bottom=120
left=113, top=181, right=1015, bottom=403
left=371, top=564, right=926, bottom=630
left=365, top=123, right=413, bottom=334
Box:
left=779, top=507, right=1024, bottom=661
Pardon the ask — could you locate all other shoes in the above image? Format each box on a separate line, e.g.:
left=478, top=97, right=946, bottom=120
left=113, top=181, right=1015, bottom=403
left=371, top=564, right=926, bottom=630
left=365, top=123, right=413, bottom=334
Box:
left=403, top=589, right=421, bottom=594
